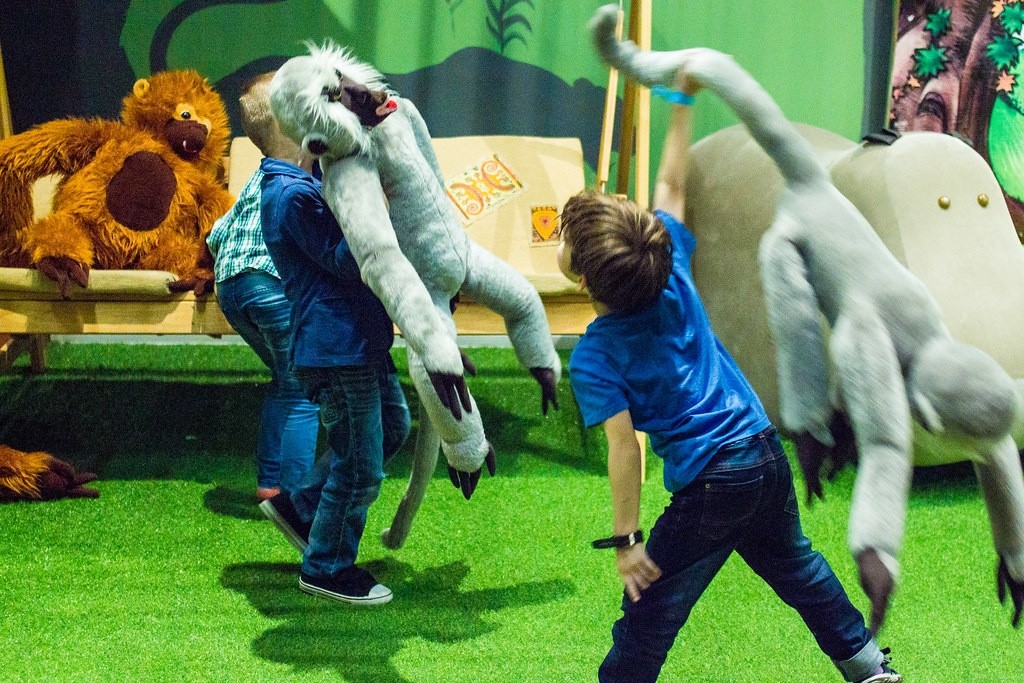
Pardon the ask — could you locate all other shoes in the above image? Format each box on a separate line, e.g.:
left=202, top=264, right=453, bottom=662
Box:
left=256, top=488, right=279, bottom=501
left=854, top=646, right=903, bottom=683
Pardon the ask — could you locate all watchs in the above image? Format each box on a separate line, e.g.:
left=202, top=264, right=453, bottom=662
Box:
left=591, top=530, right=644, bottom=551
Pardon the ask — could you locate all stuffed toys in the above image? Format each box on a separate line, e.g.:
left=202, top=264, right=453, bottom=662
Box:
left=0, top=443, right=101, bottom=504
left=589, top=4, right=1023, bottom=637
left=1, top=68, right=239, bottom=301
left=268, top=35, right=565, bottom=551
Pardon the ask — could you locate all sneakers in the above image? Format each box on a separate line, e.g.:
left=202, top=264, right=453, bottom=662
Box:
left=297, top=563, right=393, bottom=606
left=259, top=492, right=314, bottom=554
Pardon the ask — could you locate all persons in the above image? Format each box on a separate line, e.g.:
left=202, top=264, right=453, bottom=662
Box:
left=555, top=191, right=906, bottom=682
left=204, top=68, right=413, bottom=607
